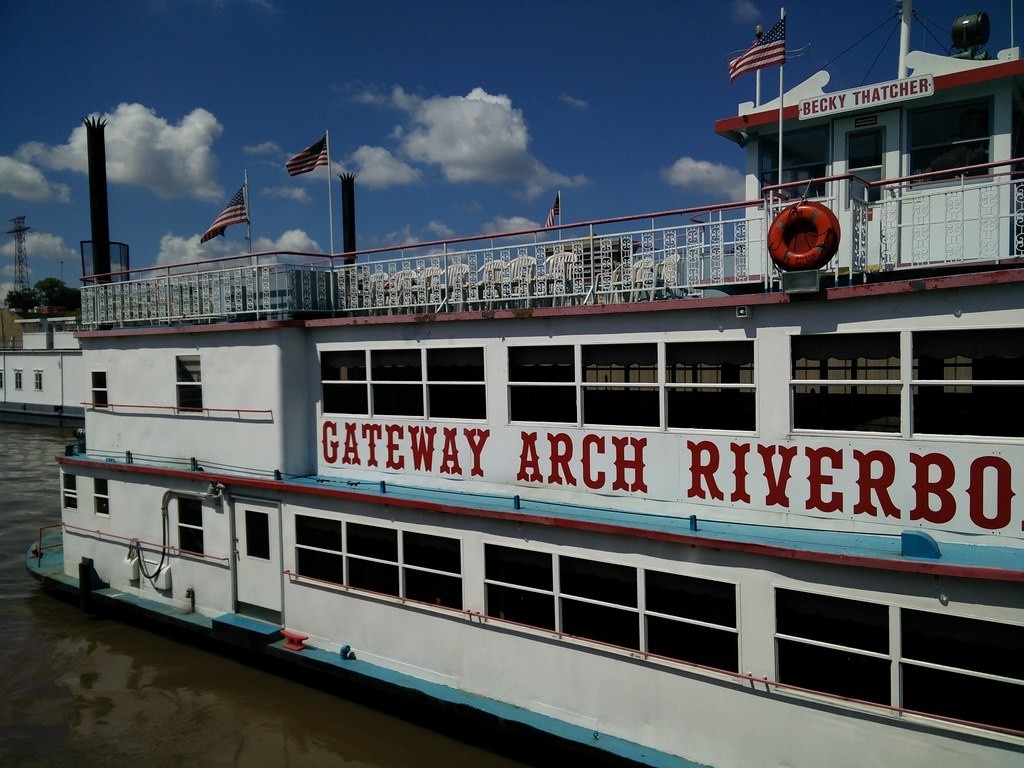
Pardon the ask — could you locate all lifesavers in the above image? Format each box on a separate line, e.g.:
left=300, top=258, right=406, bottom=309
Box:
left=767, top=198, right=835, bottom=266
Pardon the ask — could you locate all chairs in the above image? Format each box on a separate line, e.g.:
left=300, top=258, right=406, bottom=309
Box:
left=349, top=252, right=680, bottom=303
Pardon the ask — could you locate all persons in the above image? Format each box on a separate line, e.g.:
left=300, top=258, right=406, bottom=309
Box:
left=39, top=291, right=50, bottom=319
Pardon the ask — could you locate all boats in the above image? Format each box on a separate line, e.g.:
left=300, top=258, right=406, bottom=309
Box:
left=25, top=0, right=1024, bottom=768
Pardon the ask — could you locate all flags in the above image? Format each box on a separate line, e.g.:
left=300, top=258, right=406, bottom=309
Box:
left=200, top=184, right=249, bottom=244
left=727, top=15, right=787, bottom=84
left=544, top=192, right=560, bottom=233
left=285, top=133, right=328, bottom=176
